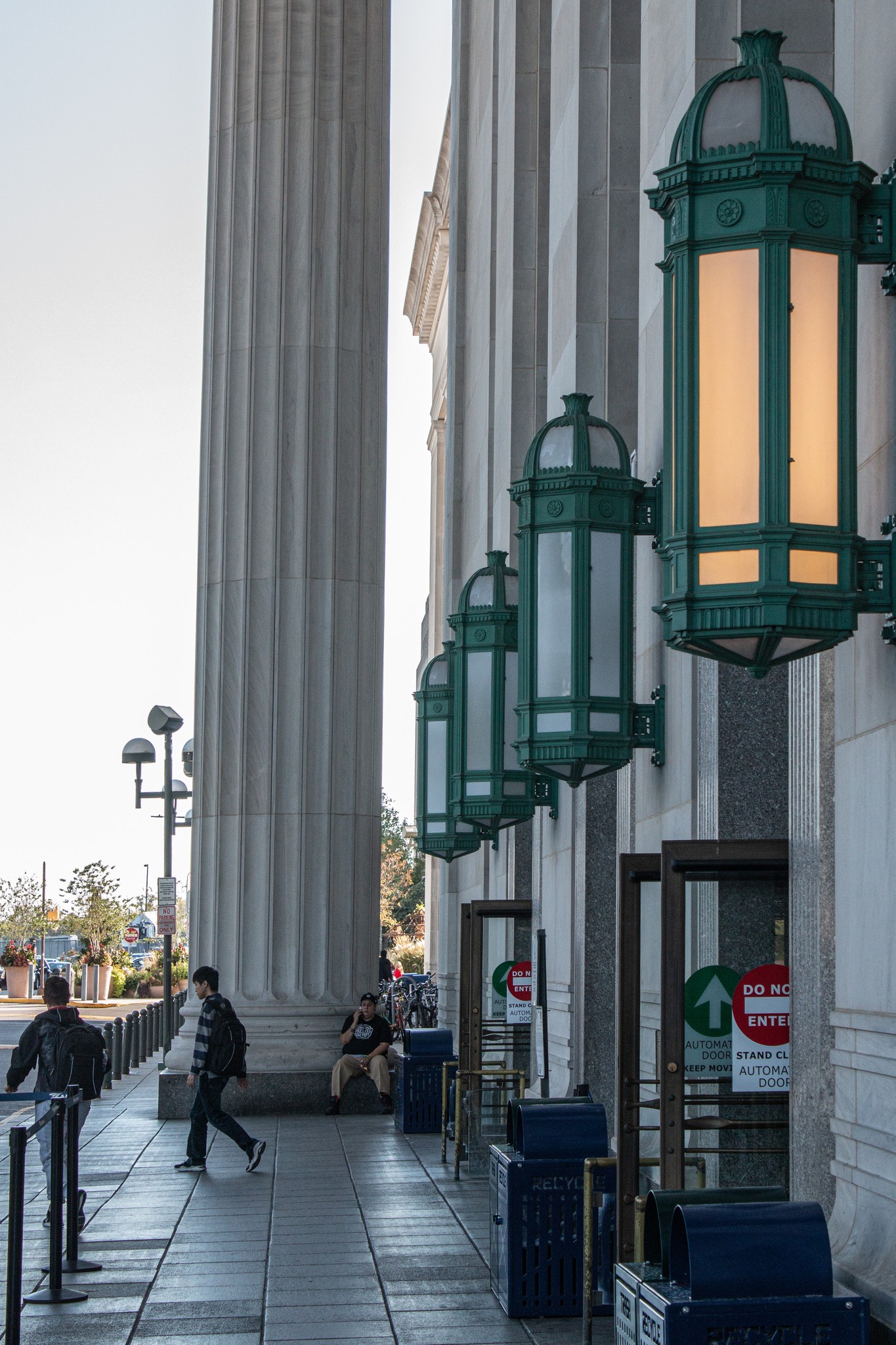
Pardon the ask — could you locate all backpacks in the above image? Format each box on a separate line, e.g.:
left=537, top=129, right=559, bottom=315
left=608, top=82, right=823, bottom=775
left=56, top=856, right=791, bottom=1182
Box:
left=46, top=1016, right=108, bottom=1101
left=202, top=998, right=247, bottom=1077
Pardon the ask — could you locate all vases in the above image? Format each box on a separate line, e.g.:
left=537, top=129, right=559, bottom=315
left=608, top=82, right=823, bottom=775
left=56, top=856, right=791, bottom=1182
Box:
left=4, top=966, right=30, bottom=999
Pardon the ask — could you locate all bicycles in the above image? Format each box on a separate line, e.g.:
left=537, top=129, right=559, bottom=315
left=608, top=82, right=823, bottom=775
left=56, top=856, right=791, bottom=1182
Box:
left=378, top=972, right=438, bottom=1042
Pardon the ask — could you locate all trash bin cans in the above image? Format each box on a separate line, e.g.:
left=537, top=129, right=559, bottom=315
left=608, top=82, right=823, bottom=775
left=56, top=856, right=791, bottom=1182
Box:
left=614, top=1185, right=868, bottom=1345
left=394, top=1028, right=459, bottom=1135
left=412, top=974, right=429, bottom=996
left=402, top=973, right=418, bottom=996
left=488, top=1096, right=618, bottom=1321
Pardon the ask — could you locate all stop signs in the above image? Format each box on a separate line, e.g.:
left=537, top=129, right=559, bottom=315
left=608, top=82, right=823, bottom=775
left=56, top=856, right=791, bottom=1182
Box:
left=121, top=927, right=139, bottom=944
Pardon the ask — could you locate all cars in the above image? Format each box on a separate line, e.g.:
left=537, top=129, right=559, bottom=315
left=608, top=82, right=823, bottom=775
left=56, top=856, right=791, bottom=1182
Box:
left=0, top=954, right=154, bottom=992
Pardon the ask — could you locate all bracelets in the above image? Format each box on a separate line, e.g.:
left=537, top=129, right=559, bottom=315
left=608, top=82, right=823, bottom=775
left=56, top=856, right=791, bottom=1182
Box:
left=365, top=1055, right=371, bottom=1061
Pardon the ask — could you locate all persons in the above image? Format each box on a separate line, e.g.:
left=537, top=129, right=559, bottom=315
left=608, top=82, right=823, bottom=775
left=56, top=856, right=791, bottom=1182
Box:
left=4, top=975, right=113, bottom=1233
left=390, top=961, right=395, bottom=975
left=324, top=992, right=393, bottom=1115
left=392, top=961, right=404, bottom=981
left=0, top=968, right=7, bottom=994
left=173, top=965, right=266, bottom=1172
left=379, top=950, right=393, bottom=996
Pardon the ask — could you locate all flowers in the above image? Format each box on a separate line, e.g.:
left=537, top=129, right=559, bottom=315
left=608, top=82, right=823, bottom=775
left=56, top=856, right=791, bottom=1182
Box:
left=0, top=940, right=38, bottom=968
left=78, top=943, right=188, bottom=1000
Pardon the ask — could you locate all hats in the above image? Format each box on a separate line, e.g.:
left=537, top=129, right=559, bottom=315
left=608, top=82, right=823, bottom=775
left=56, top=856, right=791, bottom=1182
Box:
left=360, top=993, right=376, bottom=1004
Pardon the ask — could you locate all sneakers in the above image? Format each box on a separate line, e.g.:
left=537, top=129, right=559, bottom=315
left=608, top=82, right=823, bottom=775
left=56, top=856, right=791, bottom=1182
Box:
left=42, top=1203, right=64, bottom=1227
left=246, top=1138, right=266, bottom=1172
left=77, top=1189, right=87, bottom=1232
left=174, top=1157, right=207, bottom=1171
left=380, top=1094, right=394, bottom=1114
left=325, top=1095, right=339, bottom=1115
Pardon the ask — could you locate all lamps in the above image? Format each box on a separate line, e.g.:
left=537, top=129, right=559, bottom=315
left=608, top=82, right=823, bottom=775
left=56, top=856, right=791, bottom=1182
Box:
left=508, top=391, right=668, bottom=788
left=411, top=641, right=500, bottom=863
left=646, top=28, right=896, bottom=680
left=445, top=550, right=560, bottom=836
